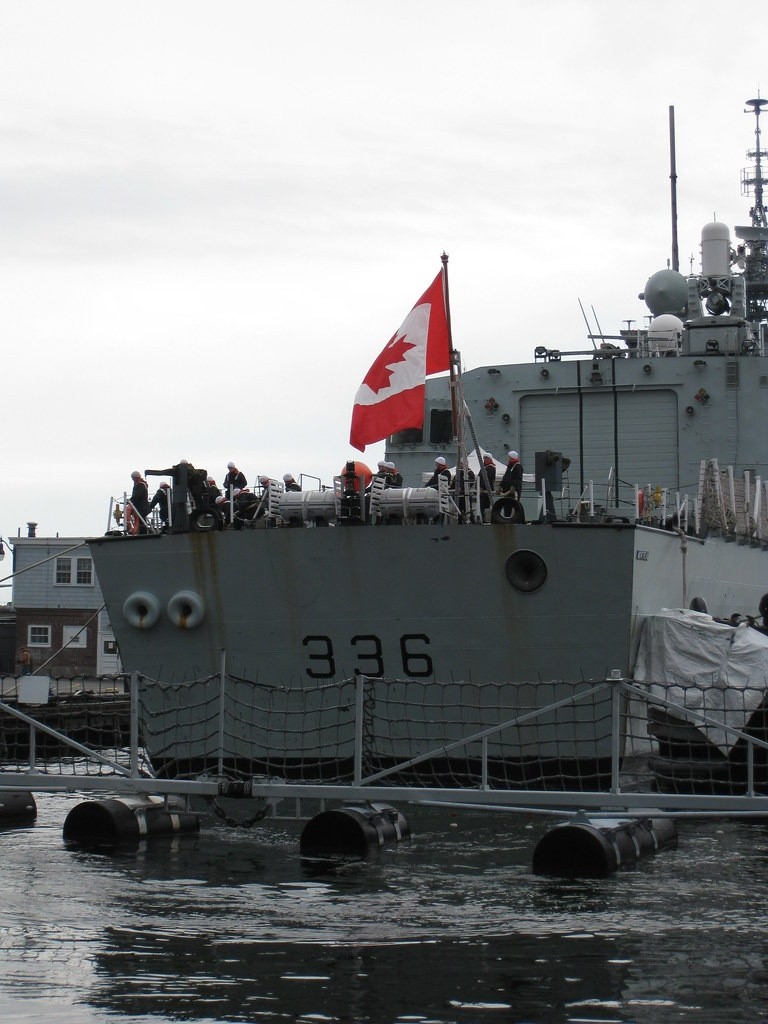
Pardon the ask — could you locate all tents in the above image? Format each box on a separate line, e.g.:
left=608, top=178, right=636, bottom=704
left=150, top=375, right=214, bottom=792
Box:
left=422, top=446, right=535, bottom=483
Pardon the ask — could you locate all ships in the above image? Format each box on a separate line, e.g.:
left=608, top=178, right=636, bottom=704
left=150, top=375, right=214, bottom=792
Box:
left=78, top=86, right=767, bottom=778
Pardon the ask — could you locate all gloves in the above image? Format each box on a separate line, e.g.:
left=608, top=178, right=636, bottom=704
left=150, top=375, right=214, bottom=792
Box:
left=496, top=485, right=501, bottom=494
left=510, top=485, right=516, bottom=494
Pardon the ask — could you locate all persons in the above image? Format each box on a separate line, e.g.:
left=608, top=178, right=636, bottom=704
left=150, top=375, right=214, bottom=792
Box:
left=216, top=489, right=264, bottom=529
left=259, top=475, right=280, bottom=527
left=365, top=461, right=386, bottom=494
left=496, top=451, right=523, bottom=517
left=149, top=482, right=170, bottom=522
left=478, top=453, right=496, bottom=520
left=451, top=457, right=475, bottom=523
left=384, top=462, right=403, bottom=488
left=283, top=472, right=301, bottom=492
left=130, top=471, right=150, bottom=534
left=145, top=459, right=221, bottom=513
left=424, top=457, right=451, bottom=523
left=223, top=462, right=247, bottom=499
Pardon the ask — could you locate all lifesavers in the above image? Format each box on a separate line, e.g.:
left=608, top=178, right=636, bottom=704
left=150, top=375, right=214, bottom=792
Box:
left=189, top=506, right=224, bottom=531
left=126, top=503, right=139, bottom=534
left=493, top=498, right=525, bottom=523
left=638, top=488, right=650, bottom=516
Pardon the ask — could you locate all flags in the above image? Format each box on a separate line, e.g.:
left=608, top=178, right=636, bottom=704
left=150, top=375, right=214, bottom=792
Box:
left=350, top=270, right=450, bottom=453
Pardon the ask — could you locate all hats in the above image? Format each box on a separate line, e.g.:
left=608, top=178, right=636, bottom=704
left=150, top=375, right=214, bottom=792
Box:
left=181, top=459, right=188, bottom=464
left=283, top=474, right=293, bottom=481
left=378, top=460, right=387, bottom=467
left=384, top=462, right=395, bottom=469
left=508, top=451, right=519, bottom=459
left=208, top=477, right=213, bottom=482
left=132, top=471, right=141, bottom=478
left=227, top=462, right=235, bottom=468
left=460, top=457, right=468, bottom=466
left=215, top=496, right=225, bottom=504
left=160, top=482, right=167, bottom=487
left=435, top=457, right=446, bottom=466
left=258, top=476, right=268, bottom=483
left=483, top=453, right=493, bottom=460
left=233, top=489, right=241, bottom=497
